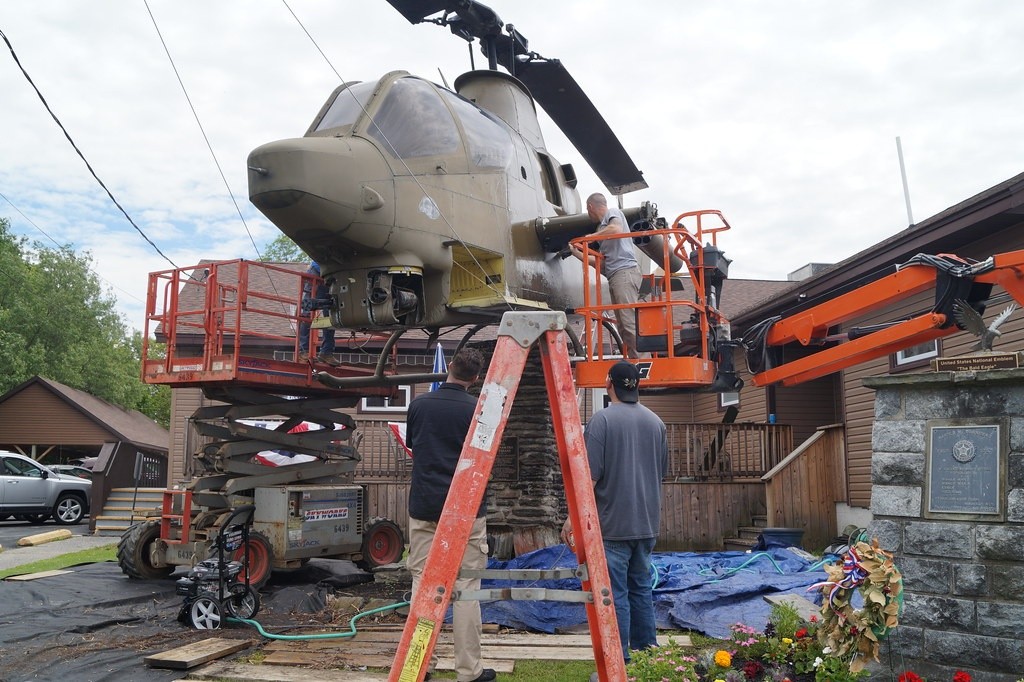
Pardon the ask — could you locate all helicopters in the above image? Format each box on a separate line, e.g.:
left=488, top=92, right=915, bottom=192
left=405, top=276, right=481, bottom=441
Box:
left=246, top=0, right=683, bottom=390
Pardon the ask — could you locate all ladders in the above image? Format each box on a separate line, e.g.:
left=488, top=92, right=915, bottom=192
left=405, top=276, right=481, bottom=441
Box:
left=386, top=311, right=628, bottom=681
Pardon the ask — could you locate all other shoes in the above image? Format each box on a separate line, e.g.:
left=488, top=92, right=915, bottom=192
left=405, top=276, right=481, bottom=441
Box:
left=457, top=668, right=496, bottom=682
left=424, top=672, right=431, bottom=681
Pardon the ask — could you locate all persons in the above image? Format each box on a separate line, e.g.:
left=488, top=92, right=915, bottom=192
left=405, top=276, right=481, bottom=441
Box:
left=405, top=348, right=496, bottom=682
left=559, top=361, right=670, bottom=682
left=295, top=261, right=343, bottom=366
left=568, top=193, right=644, bottom=358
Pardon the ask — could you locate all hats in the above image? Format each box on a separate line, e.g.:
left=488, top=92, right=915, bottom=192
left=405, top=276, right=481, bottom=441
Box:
left=609, top=359, right=640, bottom=402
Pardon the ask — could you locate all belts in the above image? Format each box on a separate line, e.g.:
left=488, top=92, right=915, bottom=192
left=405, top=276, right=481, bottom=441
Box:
left=311, top=266, right=316, bottom=272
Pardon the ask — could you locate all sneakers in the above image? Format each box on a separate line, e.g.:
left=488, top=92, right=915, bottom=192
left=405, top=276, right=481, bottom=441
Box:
left=317, top=352, right=340, bottom=365
left=292, top=351, right=319, bottom=362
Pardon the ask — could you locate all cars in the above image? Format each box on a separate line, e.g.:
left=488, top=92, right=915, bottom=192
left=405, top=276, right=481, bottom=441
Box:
left=23, top=464, right=93, bottom=518
left=0, top=450, right=93, bottom=525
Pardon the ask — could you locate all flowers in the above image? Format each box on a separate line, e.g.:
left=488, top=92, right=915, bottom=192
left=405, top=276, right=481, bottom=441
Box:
left=724, top=622, right=762, bottom=659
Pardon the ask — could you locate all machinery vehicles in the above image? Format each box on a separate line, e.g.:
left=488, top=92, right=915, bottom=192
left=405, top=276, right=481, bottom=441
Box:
left=116, top=257, right=406, bottom=589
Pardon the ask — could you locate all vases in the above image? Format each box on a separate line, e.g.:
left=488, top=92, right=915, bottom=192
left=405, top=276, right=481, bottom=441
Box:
left=744, top=659, right=760, bottom=679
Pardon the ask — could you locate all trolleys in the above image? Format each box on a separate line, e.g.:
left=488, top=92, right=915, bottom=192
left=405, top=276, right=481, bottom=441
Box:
left=176, top=505, right=260, bottom=630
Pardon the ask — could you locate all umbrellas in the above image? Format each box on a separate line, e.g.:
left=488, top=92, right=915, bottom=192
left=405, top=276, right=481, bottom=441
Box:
left=428, top=342, right=448, bottom=394
